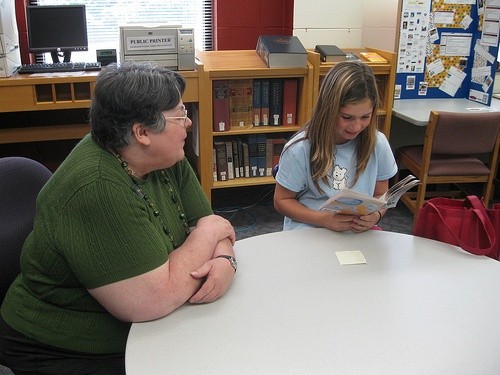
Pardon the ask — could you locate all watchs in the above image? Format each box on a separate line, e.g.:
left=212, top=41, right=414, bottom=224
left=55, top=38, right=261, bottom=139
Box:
left=216, top=255, right=236, bottom=272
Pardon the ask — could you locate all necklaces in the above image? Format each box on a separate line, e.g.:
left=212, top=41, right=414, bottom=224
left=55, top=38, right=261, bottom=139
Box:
left=109, top=148, right=190, bottom=251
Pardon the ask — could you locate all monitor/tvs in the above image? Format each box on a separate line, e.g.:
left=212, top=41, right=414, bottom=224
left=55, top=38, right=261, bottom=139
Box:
left=26, top=5, right=88, bottom=62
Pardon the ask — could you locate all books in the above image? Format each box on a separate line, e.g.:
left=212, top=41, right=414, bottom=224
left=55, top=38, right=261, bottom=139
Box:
left=315, top=45, right=388, bottom=64
left=212, top=79, right=297, bottom=132
left=212, top=136, right=288, bottom=182
left=256, top=36, right=307, bottom=68
left=320, top=174, right=422, bottom=217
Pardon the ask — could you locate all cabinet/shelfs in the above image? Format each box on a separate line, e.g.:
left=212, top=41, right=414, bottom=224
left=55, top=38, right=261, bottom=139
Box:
left=196, top=47, right=397, bottom=208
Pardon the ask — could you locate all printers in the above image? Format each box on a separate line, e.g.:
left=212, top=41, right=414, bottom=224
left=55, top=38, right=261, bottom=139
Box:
left=119, top=24, right=195, bottom=71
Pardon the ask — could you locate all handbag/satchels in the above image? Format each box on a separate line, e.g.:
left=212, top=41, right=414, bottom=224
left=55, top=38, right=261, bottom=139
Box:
left=414, top=196, right=500, bottom=261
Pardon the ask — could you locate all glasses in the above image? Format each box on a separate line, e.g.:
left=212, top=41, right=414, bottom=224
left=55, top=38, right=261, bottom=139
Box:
left=165, top=105, right=188, bottom=128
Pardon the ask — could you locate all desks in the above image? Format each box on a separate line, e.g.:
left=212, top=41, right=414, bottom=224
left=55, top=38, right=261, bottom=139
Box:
left=393, top=97, right=500, bottom=126
left=0, top=59, right=204, bottom=144
left=125, top=227, right=500, bottom=375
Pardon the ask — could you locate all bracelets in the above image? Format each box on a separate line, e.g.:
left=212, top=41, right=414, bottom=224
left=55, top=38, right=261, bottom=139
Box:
left=375, top=210, right=381, bottom=225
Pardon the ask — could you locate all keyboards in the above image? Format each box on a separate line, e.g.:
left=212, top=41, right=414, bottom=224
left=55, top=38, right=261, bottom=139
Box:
left=18, top=62, right=101, bottom=74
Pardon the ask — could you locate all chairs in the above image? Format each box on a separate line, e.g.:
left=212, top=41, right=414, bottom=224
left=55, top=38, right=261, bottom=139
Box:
left=0, top=157, right=53, bottom=305
left=394, top=112, right=500, bottom=226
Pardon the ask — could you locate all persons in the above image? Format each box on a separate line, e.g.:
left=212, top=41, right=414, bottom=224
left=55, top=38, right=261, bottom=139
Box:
left=0, top=64, right=237, bottom=375
left=274, top=61, right=398, bottom=231
